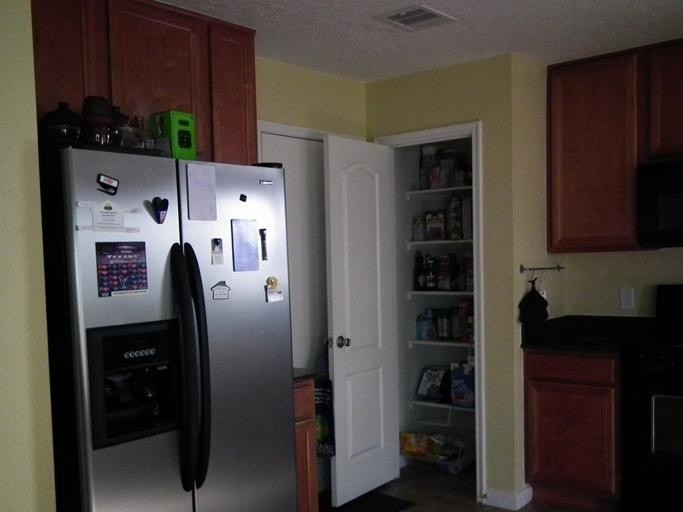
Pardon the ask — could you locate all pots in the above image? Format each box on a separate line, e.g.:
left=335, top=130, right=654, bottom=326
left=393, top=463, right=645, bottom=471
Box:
left=39, top=95, right=129, bottom=150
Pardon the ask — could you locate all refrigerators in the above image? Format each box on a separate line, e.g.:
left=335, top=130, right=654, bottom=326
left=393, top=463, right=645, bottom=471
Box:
left=47, top=147, right=296, bottom=511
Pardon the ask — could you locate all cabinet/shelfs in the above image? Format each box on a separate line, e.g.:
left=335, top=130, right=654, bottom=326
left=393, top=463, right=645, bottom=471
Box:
left=545, top=49, right=642, bottom=253
left=524, top=352, right=623, bottom=512
left=31, top=0, right=259, bottom=165
left=322, top=123, right=481, bottom=512
left=642, top=38, right=683, bottom=165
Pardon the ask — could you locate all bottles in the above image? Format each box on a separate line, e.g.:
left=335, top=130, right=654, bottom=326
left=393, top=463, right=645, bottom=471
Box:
left=413, top=248, right=473, bottom=293
left=131, top=115, right=160, bottom=147
left=416, top=299, right=473, bottom=343
